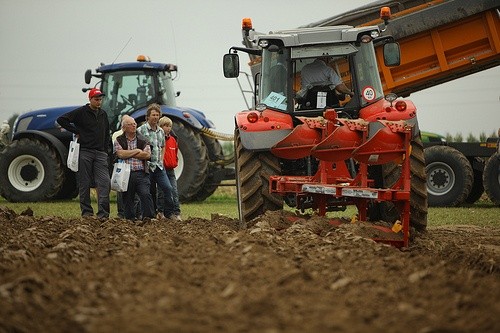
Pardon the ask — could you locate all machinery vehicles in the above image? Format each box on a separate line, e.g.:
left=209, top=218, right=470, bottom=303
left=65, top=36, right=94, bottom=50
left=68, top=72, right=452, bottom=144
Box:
left=0, top=0, right=500, bottom=248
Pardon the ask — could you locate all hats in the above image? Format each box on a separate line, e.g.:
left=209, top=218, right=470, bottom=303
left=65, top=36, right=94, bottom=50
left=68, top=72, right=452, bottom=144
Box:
left=88, top=88, right=106, bottom=99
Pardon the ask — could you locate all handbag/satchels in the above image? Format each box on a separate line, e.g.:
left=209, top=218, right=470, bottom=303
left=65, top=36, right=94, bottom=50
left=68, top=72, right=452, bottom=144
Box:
left=67, top=137, right=81, bottom=172
left=110, top=159, right=131, bottom=192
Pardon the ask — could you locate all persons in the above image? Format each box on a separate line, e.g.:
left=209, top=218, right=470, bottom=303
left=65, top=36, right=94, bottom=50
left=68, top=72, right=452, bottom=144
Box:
left=57, top=89, right=113, bottom=220
left=136, top=104, right=179, bottom=220
left=112, top=115, right=143, bottom=221
left=113, top=118, right=156, bottom=221
left=157, top=116, right=182, bottom=221
left=296, top=56, right=355, bottom=99
